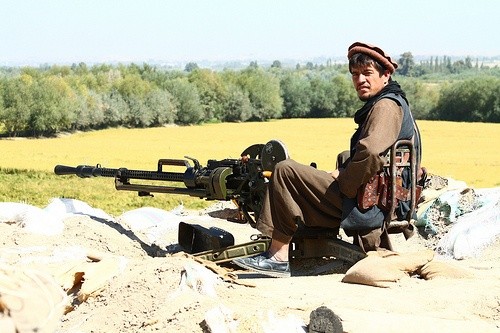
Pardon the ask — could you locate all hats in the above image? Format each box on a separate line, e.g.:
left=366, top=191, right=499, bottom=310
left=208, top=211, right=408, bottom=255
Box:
left=348, top=41, right=398, bottom=73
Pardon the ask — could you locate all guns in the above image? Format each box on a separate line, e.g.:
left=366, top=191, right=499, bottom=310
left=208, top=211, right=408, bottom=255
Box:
left=53, top=139, right=318, bottom=213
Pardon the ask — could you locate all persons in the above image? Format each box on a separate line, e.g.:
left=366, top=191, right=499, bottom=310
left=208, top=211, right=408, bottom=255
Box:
left=232, top=41, right=422, bottom=278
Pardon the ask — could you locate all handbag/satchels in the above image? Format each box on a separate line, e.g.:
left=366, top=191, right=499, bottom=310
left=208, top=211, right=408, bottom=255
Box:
left=357, top=173, right=403, bottom=209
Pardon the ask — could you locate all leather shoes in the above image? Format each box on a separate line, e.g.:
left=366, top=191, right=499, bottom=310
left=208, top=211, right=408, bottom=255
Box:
left=232, top=249, right=291, bottom=277
